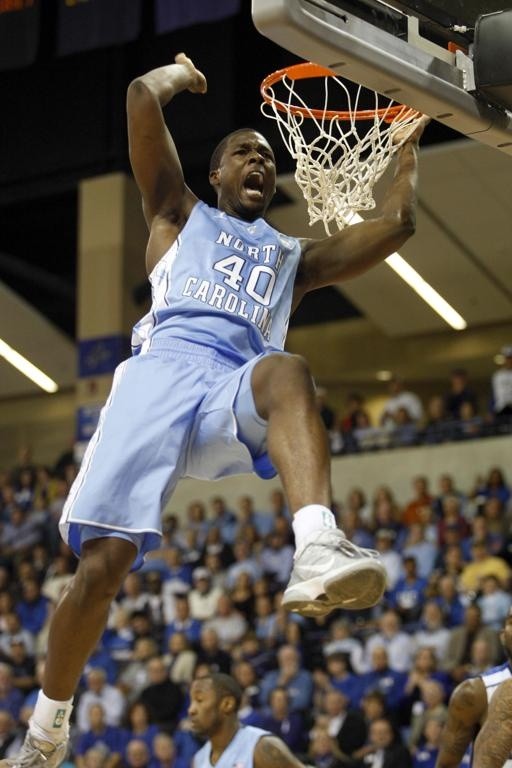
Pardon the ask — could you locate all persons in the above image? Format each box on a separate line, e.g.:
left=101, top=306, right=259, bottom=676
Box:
left=317, top=345, right=511, bottom=452
left=0, top=443, right=512, bottom=768
left=0, top=53, right=512, bottom=768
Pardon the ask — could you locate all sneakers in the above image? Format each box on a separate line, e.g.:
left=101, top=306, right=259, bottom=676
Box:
left=0, top=730, right=72, bottom=768
left=281, top=528, right=388, bottom=618
